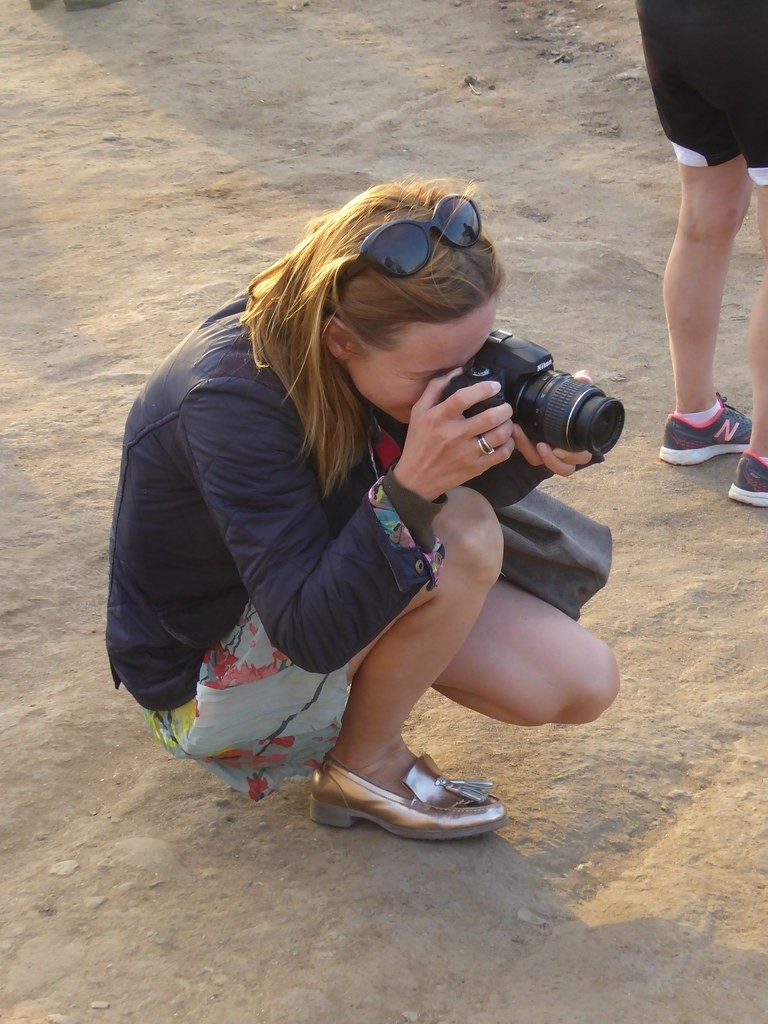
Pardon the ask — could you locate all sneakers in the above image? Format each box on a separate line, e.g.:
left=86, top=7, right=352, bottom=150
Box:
left=728, top=450, right=768, bottom=507
left=658, top=392, right=752, bottom=465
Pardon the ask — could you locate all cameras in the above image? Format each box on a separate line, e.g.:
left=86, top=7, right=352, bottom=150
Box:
left=439, top=330, right=625, bottom=455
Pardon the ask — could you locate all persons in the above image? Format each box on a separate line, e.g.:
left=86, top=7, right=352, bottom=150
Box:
left=106, top=179, right=620, bottom=841
left=633, top=0, right=768, bottom=510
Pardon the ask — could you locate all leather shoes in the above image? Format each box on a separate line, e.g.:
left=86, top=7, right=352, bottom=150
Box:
left=310, top=751, right=511, bottom=839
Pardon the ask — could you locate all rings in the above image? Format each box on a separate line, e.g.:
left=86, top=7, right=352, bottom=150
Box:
left=478, top=434, right=494, bottom=455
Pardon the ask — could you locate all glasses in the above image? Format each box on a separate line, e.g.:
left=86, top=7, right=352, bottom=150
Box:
left=340, top=194, right=482, bottom=288
